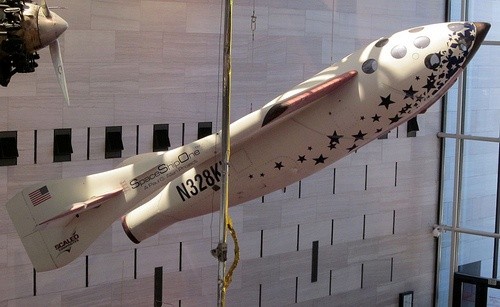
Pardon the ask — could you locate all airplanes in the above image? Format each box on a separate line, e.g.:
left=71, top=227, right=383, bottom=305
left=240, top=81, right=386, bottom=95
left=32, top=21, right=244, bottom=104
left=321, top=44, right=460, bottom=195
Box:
left=0, top=0, right=72, bottom=108
left=4, top=21, right=492, bottom=275
